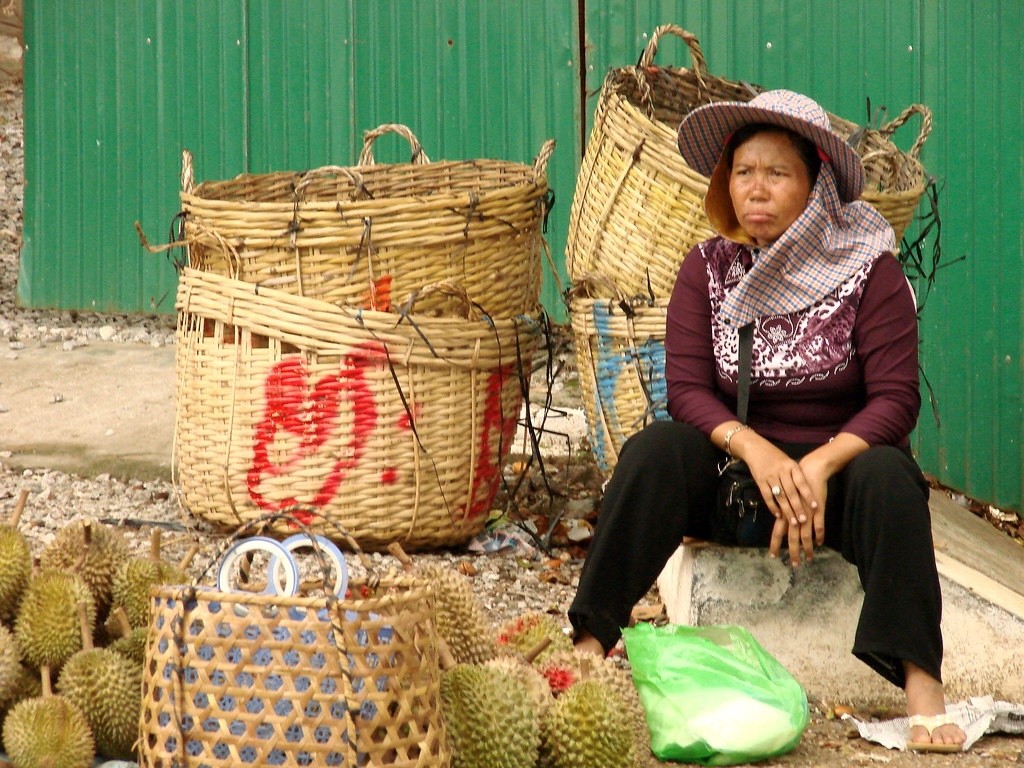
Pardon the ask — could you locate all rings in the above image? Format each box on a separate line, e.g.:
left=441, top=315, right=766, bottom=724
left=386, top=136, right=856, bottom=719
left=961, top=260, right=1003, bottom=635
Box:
left=772, top=486, right=781, bottom=496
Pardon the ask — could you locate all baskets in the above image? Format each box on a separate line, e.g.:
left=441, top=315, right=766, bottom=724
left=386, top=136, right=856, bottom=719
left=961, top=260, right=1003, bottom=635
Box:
left=164, top=130, right=553, bottom=547
left=139, top=533, right=450, bottom=768
left=565, top=23, right=932, bottom=481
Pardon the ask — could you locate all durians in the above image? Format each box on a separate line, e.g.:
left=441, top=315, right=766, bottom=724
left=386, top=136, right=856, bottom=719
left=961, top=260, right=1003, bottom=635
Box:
left=402, top=558, right=652, bottom=768
left=0, top=489, right=199, bottom=768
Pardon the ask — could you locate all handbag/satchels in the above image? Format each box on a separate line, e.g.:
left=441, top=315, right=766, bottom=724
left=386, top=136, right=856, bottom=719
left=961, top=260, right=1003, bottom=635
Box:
left=620, top=623, right=810, bottom=765
left=715, top=447, right=843, bottom=525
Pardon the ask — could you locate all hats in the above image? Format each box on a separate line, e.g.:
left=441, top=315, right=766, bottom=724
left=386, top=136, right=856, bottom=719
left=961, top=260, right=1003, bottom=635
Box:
left=678, top=90, right=866, bottom=204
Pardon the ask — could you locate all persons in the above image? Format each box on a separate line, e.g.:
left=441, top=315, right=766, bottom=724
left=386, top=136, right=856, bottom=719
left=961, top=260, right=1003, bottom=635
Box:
left=565, top=89, right=968, bottom=752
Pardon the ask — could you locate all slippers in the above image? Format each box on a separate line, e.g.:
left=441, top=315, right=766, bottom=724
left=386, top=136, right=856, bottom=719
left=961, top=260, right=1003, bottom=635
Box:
left=909, top=715, right=964, bottom=751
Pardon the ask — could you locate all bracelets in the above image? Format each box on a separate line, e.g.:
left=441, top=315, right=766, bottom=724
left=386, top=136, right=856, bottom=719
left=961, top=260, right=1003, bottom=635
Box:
left=724, top=425, right=755, bottom=456
